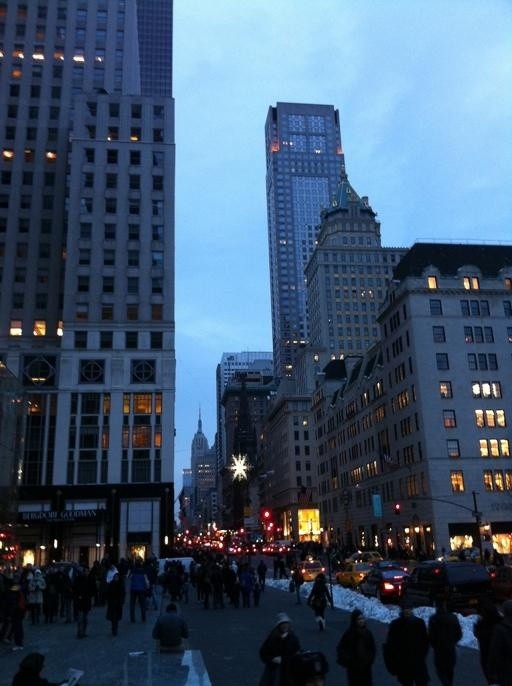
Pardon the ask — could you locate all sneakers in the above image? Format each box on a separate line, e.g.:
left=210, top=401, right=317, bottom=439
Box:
left=197, top=598, right=259, bottom=610
left=110, top=629, right=119, bottom=637
left=77, top=631, right=87, bottom=638
left=130, top=618, right=146, bottom=624
left=2, top=636, right=24, bottom=652
left=28, top=615, right=74, bottom=627
left=146, top=597, right=192, bottom=612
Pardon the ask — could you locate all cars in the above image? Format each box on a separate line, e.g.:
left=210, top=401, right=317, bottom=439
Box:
left=179, top=535, right=291, bottom=555
left=296, top=550, right=511, bottom=615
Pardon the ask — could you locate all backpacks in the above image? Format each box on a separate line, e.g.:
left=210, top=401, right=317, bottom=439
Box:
left=297, top=573, right=304, bottom=585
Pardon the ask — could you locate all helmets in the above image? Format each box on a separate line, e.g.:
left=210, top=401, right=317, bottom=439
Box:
left=286, top=649, right=330, bottom=680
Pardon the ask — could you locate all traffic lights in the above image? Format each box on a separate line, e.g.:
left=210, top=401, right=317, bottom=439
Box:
left=393, top=503, right=401, bottom=514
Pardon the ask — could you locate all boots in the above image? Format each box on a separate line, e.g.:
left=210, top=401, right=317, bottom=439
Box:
left=316, top=616, right=327, bottom=630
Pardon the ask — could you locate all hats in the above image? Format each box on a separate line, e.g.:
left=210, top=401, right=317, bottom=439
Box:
left=274, top=611, right=291, bottom=628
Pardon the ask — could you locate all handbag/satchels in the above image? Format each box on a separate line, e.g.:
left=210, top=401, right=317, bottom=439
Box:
left=380, top=643, right=398, bottom=677
left=288, top=582, right=295, bottom=593
